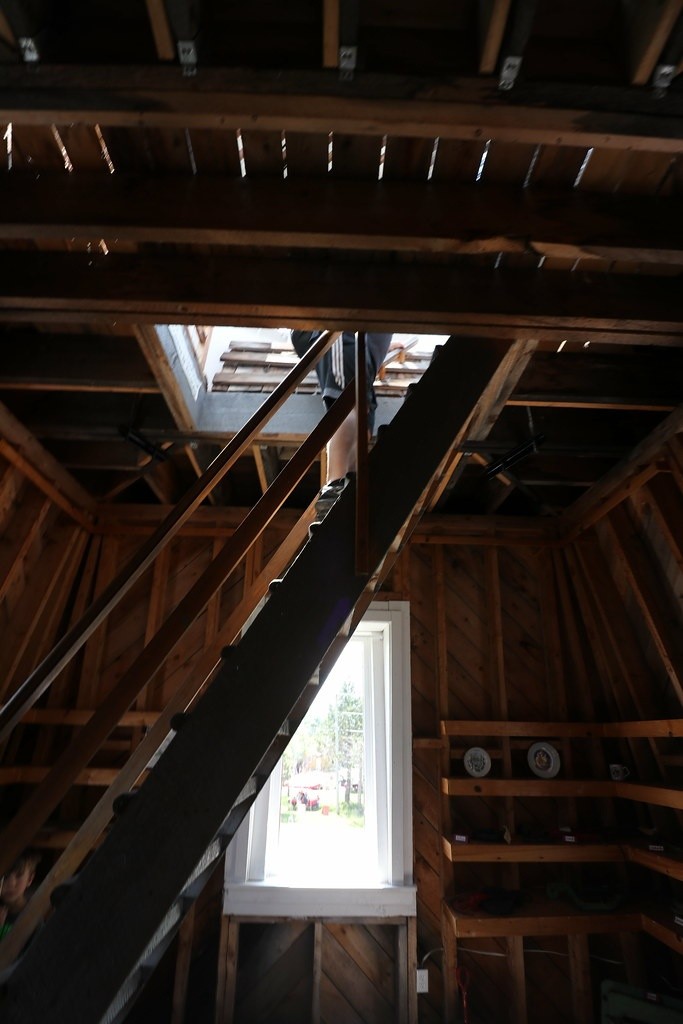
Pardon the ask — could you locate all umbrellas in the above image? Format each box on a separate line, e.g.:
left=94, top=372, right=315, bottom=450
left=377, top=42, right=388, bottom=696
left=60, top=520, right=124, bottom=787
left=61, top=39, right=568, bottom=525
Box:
left=283, top=770, right=338, bottom=791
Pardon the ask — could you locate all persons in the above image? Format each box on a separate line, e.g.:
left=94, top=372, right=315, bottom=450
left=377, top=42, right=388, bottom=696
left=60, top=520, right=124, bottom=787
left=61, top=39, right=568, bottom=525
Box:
left=290, top=791, right=308, bottom=811
left=290, top=328, right=395, bottom=536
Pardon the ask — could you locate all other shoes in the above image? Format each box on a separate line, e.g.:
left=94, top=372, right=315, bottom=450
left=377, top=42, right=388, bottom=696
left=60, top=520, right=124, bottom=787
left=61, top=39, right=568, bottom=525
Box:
left=315, top=479, right=344, bottom=522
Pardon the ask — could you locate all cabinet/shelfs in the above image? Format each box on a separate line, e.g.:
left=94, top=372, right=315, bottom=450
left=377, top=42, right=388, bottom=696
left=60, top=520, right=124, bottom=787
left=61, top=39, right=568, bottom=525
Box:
left=439, top=719, right=683, bottom=954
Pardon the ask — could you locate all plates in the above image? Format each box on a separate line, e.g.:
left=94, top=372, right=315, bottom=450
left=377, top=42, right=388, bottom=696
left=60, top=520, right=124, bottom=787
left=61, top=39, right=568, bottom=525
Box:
left=464, top=746, right=492, bottom=777
left=528, top=742, right=561, bottom=780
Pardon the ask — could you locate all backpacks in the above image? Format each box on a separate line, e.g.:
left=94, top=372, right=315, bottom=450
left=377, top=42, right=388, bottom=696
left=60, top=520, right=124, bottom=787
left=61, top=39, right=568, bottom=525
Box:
left=292, top=328, right=324, bottom=358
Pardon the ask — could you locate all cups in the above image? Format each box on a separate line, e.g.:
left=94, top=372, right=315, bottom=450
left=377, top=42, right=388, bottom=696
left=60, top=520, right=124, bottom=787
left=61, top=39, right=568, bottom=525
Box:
left=609, top=764, right=629, bottom=782
